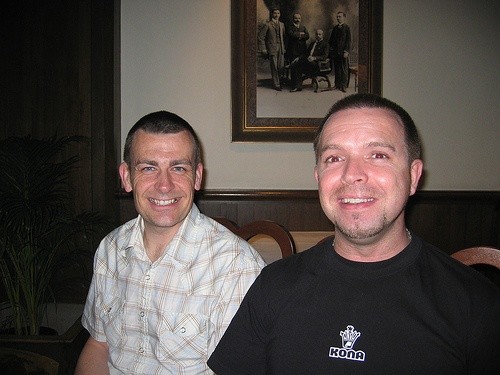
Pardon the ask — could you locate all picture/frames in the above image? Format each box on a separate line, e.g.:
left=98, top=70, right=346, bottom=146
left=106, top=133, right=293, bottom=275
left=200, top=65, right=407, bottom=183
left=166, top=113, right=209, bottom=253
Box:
left=231, top=0, right=383, bottom=142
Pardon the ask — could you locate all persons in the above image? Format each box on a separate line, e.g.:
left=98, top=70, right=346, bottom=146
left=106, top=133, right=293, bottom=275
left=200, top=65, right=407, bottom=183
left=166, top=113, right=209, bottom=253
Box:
left=73, top=110, right=267, bottom=375
left=205, top=93, right=500, bottom=375
left=260, top=8, right=352, bottom=92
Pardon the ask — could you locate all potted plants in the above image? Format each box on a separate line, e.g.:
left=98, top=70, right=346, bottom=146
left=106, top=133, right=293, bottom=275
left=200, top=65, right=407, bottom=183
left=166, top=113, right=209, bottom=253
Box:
left=0, top=129, right=120, bottom=375
